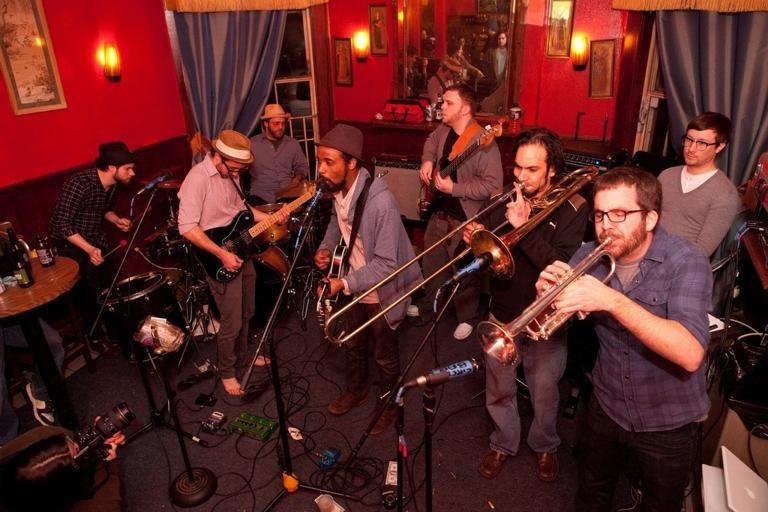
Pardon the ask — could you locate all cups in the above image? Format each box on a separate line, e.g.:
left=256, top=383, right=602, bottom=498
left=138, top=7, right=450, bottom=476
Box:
left=316, top=494, right=334, bottom=511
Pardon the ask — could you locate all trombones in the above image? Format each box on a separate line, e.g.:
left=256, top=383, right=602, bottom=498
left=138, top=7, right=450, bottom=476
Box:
left=324, top=164, right=600, bottom=346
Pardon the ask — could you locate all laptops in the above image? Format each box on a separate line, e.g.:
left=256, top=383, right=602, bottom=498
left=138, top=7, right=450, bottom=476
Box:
left=701, top=444, right=768, bottom=512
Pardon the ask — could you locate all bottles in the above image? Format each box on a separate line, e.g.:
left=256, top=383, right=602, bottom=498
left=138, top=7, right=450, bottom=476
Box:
left=507, top=103, right=522, bottom=134
left=426, top=92, right=444, bottom=123
left=34, top=231, right=56, bottom=267
left=6, top=225, right=36, bottom=288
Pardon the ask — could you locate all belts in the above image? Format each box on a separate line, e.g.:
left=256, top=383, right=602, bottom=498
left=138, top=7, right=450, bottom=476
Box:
left=432, top=211, right=454, bottom=247
left=487, top=314, right=505, bottom=328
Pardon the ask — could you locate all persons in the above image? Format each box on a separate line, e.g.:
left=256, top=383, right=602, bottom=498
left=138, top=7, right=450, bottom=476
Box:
left=311, top=122, right=425, bottom=419
left=46, top=140, right=139, bottom=368
left=405, top=83, right=505, bottom=341
left=0, top=414, right=129, bottom=511
left=0, top=314, right=65, bottom=428
left=176, top=127, right=292, bottom=396
left=534, top=167, right=715, bottom=511
left=657, top=111, right=739, bottom=258
left=246, top=100, right=309, bottom=207
left=400, top=26, right=508, bottom=104
left=461, top=126, right=590, bottom=482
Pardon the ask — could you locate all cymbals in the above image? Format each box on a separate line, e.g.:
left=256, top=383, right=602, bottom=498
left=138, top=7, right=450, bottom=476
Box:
left=141, top=175, right=184, bottom=191
left=277, top=183, right=326, bottom=201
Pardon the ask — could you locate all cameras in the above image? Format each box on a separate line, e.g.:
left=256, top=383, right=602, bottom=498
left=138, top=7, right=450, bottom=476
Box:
left=75, top=402, right=137, bottom=472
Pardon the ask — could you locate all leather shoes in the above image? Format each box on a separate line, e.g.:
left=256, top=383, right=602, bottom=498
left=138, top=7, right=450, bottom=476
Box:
left=88, top=339, right=123, bottom=361
left=476, top=447, right=509, bottom=480
left=535, top=450, right=560, bottom=482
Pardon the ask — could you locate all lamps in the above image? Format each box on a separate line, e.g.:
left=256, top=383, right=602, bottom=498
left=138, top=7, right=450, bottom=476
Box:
left=350, top=29, right=371, bottom=64
left=99, top=40, right=125, bottom=85
left=568, top=30, right=589, bottom=72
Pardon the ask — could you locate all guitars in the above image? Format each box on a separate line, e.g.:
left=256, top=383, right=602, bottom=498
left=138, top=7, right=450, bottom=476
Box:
left=417, top=120, right=504, bottom=219
left=317, top=236, right=348, bottom=339
left=196, top=183, right=316, bottom=282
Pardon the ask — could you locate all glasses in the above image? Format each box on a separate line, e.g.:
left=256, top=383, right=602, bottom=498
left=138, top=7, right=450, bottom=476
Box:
left=680, top=134, right=721, bottom=151
left=221, top=158, right=249, bottom=175
left=587, top=208, right=651, bottom=223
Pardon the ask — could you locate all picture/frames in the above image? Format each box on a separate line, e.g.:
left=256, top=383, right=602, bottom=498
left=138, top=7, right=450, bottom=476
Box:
left=0, top=0, right=69, bottom=119
left=587, top=36, right=617, bottom=101
left=367, top=3, right=389, bottom=55
left=333, top=34, right=352, bottom=88
left=543, top=0, right=576, bottom=61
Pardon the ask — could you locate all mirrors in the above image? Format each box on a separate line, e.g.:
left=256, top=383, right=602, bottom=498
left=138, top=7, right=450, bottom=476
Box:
left=399, top=0, right=517, bottom=118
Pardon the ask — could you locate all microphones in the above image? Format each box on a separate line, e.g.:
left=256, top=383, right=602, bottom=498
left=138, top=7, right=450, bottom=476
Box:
left=404, top=356, right=483, bottom=388
left=441, top=251, right=492, bottom=284
left=301, top=179, right=328, bottom=215
left=143, top=170, right=172, bottom=189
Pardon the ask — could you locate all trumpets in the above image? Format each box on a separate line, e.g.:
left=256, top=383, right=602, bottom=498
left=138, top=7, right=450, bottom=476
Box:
left=477, top=237, right=616, bottom=368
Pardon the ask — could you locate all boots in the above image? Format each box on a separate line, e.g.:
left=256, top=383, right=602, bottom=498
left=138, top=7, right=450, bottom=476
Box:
left=327, top=377, right=372, bottom=416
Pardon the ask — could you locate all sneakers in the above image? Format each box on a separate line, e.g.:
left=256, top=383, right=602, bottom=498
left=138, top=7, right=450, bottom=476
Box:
left=25, top=381, right=56, bottom=429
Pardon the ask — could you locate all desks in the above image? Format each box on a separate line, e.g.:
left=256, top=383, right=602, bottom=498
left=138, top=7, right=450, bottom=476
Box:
left=0, top=252, right=84, bottom=434
left=331, top=115, right=527, bottom=187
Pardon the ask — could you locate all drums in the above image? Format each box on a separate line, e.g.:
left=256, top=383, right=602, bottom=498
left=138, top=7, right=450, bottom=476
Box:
left=255, top=202, right=302, bottom=245
left=252, top=246, right=293, bottom=314
left=99, top=270, right=190, bottom=363
left=141, top=223, right=192, bottom=263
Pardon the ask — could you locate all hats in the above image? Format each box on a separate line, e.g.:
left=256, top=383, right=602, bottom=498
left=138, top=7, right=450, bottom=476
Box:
left=259, top=103, right=292, bottom=124
left=312, top=123, right=364, bottom=162
left=209, top=128, right=257, bottom=165
left=93, top=141, right=138, bottom=167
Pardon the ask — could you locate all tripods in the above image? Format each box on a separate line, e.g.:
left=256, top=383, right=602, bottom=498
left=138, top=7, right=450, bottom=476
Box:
left=238, top=223, right=361, bottom=512
left=88, top=199, right=208, bottom=453
